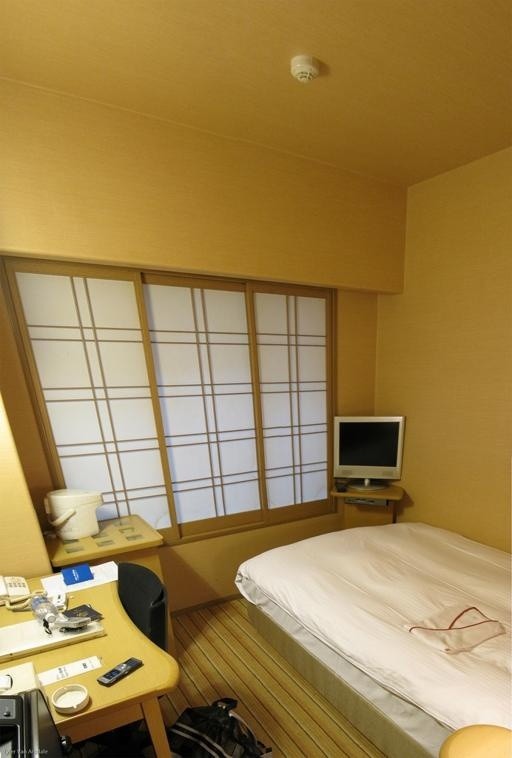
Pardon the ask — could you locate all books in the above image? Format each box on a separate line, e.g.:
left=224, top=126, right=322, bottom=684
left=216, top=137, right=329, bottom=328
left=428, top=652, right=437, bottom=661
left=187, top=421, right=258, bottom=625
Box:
left=61, top=563, right=93, bottom=585
left=40, top=561, right=118, bottom=595
left=62, top=605, right=102, bottom=622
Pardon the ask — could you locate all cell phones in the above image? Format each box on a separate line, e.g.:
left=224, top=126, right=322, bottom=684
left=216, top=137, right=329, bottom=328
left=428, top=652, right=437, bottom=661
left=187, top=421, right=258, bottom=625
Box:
left=98, top=656, right=143, bottom=686
left=52, top=592, right=67, bottom=610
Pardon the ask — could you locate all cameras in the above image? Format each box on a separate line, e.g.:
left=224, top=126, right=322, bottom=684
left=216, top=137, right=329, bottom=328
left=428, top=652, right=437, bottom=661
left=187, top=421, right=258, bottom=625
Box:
left=54, top=615, right=92, bottom=632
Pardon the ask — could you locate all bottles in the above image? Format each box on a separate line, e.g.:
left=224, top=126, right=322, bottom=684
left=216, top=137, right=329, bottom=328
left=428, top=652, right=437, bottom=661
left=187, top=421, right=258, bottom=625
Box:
left=28, top=595, right=56, bottom=624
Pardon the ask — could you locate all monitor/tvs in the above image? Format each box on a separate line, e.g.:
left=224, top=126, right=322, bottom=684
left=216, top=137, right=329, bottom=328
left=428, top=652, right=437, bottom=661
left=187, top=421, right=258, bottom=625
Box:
left=333, top=415, right=404, bottom=491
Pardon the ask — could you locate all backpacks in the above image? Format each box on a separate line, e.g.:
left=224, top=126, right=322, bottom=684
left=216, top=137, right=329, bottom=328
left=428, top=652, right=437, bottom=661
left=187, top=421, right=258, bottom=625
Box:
left=165, top=697, right=273, bottom=758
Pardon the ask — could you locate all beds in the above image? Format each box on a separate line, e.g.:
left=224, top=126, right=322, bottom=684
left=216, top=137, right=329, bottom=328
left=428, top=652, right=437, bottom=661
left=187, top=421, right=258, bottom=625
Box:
left=235, top=523, right=512, bottom=758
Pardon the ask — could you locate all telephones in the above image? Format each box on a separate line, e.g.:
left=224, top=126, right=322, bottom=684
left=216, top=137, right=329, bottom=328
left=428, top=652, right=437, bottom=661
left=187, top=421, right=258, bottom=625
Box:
left=0, top=576, right=31, bottom=605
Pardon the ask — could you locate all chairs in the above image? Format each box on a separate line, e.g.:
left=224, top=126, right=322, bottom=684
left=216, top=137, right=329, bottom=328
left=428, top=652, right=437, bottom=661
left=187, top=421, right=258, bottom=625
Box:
left=118, top=563, right=167, bottom=651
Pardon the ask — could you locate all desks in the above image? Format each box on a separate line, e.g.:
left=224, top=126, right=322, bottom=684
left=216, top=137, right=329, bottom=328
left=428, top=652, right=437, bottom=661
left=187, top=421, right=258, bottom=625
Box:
left=0, top=572, right=181, bottom=758
left=330, top=485, right=404, bottom=529
left=44, top=515, right=176, bottom=663
